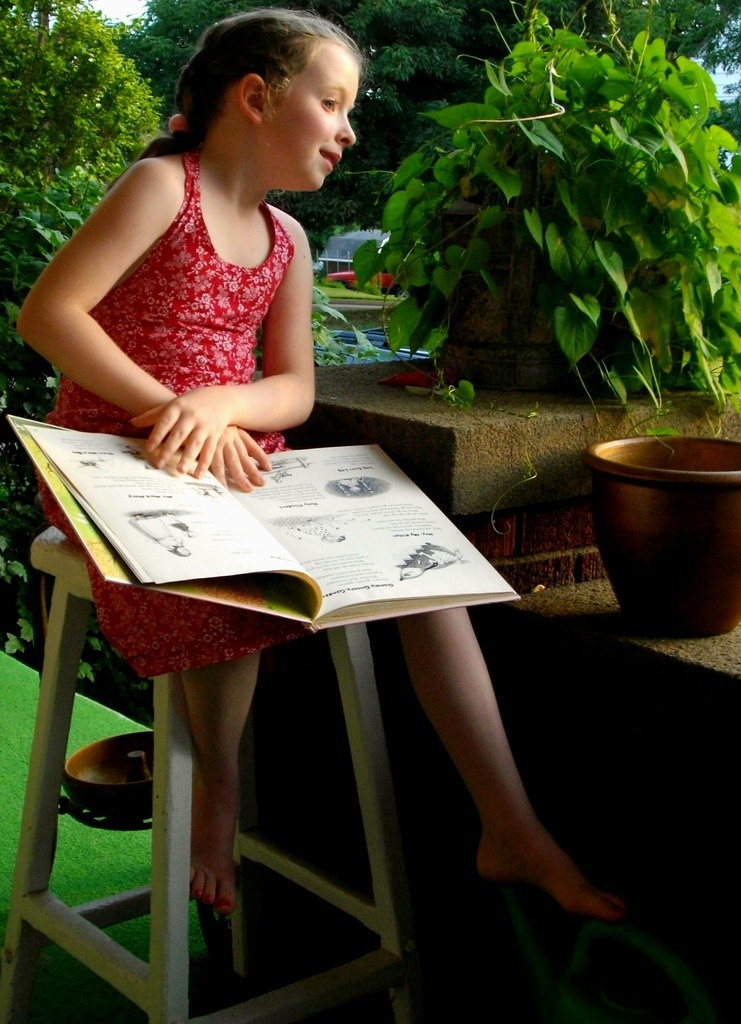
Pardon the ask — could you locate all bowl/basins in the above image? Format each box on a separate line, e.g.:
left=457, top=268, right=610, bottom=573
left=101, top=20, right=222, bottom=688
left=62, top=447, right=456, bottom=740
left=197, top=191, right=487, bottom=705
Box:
left=65, top=732, right=154, bottom=818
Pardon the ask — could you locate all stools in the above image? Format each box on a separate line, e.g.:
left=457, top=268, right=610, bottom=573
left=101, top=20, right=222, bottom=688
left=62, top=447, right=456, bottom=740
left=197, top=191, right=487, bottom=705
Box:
left=0, top=524, right=424, bottom=1024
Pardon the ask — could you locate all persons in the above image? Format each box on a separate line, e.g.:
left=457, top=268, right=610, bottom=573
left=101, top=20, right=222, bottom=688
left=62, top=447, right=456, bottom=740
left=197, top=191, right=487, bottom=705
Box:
left=17, top=9, right=626, bottom=921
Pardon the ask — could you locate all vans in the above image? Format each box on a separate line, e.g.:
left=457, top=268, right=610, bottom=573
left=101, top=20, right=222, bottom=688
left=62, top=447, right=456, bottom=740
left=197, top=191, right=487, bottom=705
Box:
left=312, top=229, right=391, bottom=281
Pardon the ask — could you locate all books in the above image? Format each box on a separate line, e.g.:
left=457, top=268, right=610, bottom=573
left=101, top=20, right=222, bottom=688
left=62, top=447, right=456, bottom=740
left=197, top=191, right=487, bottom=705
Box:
left=7, top=411, right=523, bottom=630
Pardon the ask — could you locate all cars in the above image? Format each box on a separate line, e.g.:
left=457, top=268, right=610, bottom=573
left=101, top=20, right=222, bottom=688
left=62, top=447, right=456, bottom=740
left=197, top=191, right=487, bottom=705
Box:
left=325, top=262, right=410, bottom=300
left=312, top=324, right=431, bottom=369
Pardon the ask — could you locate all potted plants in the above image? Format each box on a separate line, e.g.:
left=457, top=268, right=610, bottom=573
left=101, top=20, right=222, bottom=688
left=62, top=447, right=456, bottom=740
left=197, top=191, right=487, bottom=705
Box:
left=354, top=0, right=741, bottom=540
